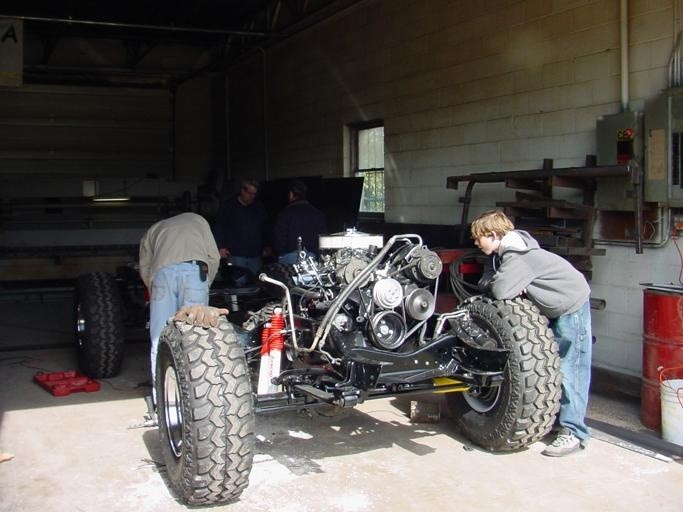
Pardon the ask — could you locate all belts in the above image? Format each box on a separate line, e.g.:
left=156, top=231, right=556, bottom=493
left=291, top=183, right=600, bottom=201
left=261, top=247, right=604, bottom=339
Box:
left=184, top=260, right=200, bottom=265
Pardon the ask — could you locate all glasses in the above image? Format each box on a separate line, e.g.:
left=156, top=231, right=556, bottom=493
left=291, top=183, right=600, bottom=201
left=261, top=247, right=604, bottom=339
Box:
left=246, top=191, right=256, bottom=195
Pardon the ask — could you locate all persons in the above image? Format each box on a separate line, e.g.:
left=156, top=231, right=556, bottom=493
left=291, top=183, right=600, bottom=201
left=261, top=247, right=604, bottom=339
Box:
left=471, top=210, right=592, bottom=456
left=272, top=181, right=331, bottom=266
left=210, top=178, right=272, bottom=287
left=139, top=212, right=221, bottom=413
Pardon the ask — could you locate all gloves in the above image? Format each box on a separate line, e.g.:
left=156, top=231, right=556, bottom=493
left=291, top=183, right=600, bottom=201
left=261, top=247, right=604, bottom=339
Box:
left=174, top=306, right=229, bottom=327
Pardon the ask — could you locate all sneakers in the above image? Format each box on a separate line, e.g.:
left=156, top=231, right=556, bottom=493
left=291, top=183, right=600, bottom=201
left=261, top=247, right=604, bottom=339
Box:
left=543, top=425, right=581, bottom=457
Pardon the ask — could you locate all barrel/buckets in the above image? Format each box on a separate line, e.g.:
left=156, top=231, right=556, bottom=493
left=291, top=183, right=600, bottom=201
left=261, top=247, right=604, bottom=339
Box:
left=642, top=290, right=683, bottom=427
left=659, top=368, right=683, bottom=445
